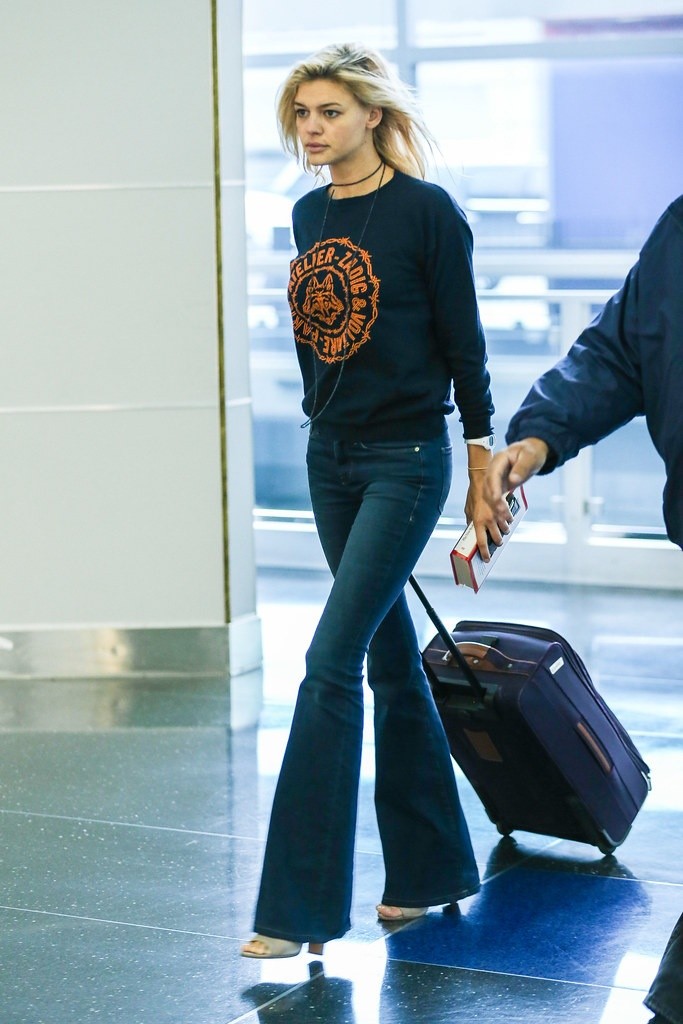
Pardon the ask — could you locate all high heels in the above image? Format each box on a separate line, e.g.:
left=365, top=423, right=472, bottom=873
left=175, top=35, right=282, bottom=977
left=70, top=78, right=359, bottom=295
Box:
left=377, top=900, right=460, bottom=920
left=240, top=933, right=327, bottom=959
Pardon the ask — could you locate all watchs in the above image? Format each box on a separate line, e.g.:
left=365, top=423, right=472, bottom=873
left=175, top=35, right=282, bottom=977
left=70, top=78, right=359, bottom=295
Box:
left=464, top=434, right=495, bottom=451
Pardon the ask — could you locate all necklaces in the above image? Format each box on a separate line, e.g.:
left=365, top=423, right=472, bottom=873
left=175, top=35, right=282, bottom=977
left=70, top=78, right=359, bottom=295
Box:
left=299, top=157, right=386, bottom=430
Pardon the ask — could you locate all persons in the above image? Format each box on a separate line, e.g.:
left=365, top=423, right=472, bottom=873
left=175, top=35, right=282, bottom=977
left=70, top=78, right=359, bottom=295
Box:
left=241, top=43, right=518, bottom=962
left=482, top=189, right=682, bottom=1021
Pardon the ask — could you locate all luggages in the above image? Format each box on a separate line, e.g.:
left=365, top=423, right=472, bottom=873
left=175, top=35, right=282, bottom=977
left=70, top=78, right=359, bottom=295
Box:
left=405, top=572, right=652, bottom=859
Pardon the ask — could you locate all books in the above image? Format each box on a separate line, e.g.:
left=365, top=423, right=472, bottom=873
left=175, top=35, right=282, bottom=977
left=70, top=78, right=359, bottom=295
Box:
left=447, top=479, right=530, bottom=593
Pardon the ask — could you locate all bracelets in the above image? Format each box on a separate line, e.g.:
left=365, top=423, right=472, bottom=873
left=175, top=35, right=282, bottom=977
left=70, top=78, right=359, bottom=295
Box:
left=468, top=467, right=487, bottom=471
